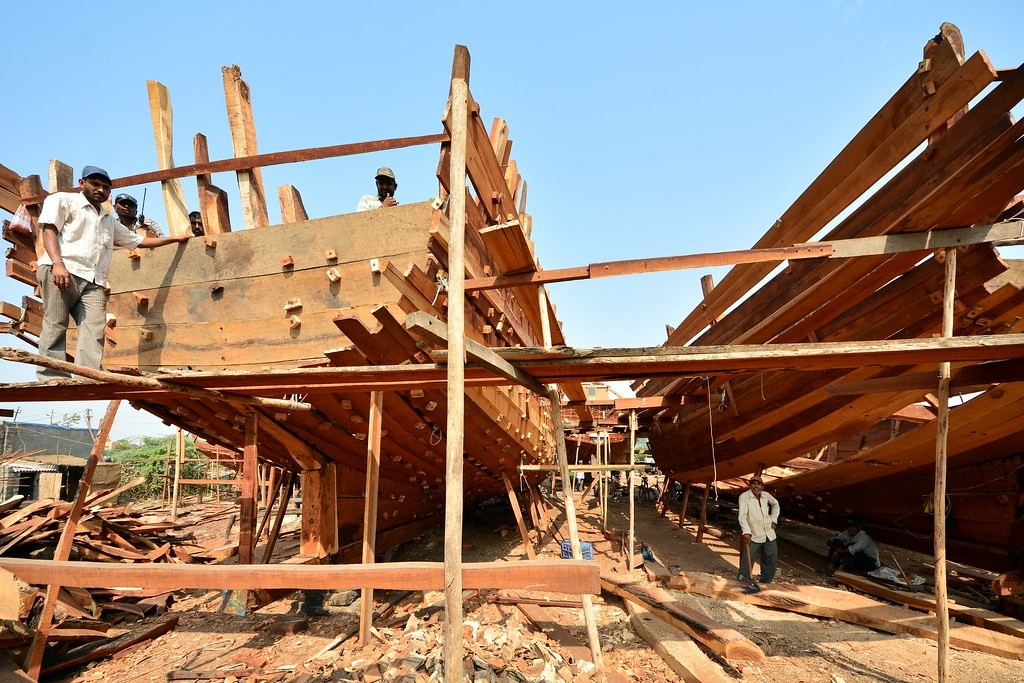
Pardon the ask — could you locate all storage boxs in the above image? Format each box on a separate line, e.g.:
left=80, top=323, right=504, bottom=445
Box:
left=561, top=540, right=593, bottom=559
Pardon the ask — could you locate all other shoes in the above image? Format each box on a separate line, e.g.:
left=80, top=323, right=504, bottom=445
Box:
left=816, top=567, right=834, bottom=576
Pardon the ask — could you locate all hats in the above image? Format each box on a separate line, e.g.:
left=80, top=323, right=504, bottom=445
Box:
left=115, top=193, right=138, bottom=206
left=375, top=167, right=395, bottom=180
left=579, top=460, right=583, bottom=463
left=82, top=166, right=112, bottom=186
left=749, top=476, right=763, bottom=483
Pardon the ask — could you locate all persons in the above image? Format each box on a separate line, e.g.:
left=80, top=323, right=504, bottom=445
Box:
left=357, top=168, right=399, bottom=212
left=189, top=211, right=206, bottom=236
left=815, top=516, right=880, bottom=577
left=35, top=166, right=193, bottom=382
left=576, top=460, right=585, bottom=492
left=738, top=476, right=780, bottom=583
left=113, top=194, right=165, bottom=239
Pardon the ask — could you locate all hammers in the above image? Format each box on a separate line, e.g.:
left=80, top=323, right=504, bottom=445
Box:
left=892, top=554, right=921, bottom=593
left=745, top=544, right=761, bottom=594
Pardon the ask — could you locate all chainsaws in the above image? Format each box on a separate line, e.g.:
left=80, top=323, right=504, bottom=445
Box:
left=640, top=542, right=655, bottom=563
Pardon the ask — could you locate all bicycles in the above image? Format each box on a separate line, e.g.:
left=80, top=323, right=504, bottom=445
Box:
left=648, top=476, right=680, bottom=503
left=639, top=476, right=649, bottom=505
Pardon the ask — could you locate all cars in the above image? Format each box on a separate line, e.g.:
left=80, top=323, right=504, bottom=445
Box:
left=644, top=455, right=655, bottom=463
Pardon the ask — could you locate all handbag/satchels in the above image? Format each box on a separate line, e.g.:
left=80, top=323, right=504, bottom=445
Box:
left=9, top=204, right=32, bottom=238
